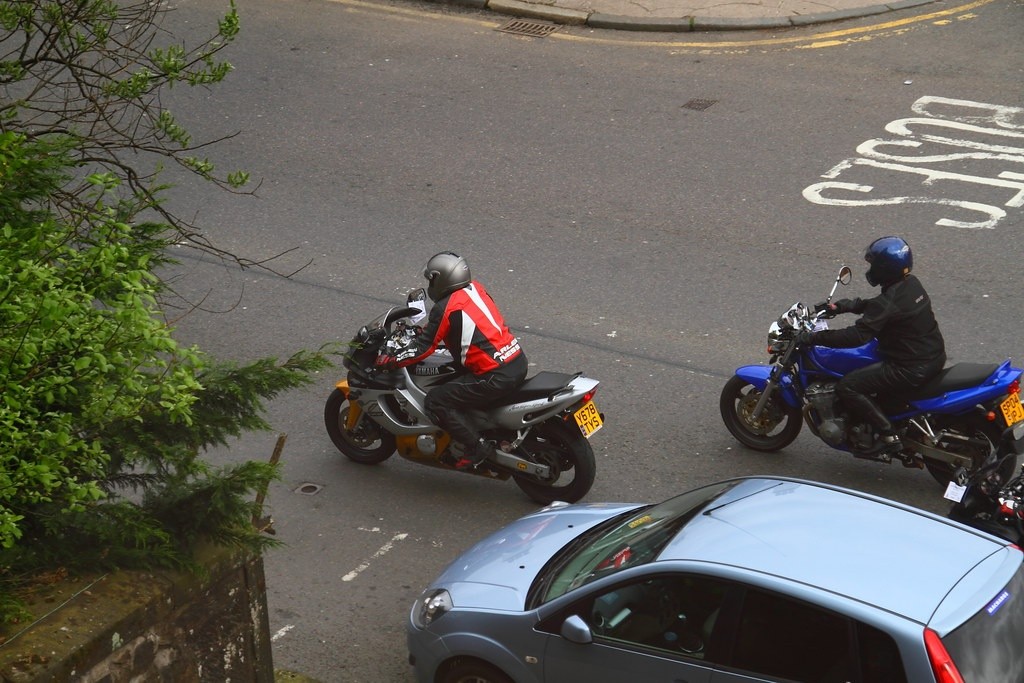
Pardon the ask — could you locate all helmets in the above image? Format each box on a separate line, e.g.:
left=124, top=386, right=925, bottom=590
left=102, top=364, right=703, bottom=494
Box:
left=865, top=236, right=912, bottom=287
left=424, top=251, right=471, bottom=303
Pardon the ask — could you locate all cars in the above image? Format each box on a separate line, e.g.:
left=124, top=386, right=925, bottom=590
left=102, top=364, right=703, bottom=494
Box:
left=406, top=474, right=1024, bottom=683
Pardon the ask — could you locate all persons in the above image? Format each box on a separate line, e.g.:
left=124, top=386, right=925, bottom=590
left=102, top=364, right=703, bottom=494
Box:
left=794, top=236, right=947, bottom=458
left=369, top=251, right=528, bottom=471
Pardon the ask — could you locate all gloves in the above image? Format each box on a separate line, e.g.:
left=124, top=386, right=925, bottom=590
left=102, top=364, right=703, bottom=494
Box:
left=376, top=354, right=399, bottom=374
left=834, top=299, right=853, bottom=314
left=795, top=331, right=819, bottom=353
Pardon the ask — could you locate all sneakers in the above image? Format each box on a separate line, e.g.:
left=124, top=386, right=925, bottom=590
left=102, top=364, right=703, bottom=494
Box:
left=455, top=442, right=492, bottom=471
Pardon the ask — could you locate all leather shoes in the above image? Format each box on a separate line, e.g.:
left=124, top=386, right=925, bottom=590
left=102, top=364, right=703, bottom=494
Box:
left=854, top=434, right=904, bottom=457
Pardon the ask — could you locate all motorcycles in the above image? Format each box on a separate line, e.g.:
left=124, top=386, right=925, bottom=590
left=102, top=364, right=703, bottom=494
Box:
left=324, top=288, right=605, bottom=505
left=720, top=266, right=1024, bottom=488
left=947, top=419, right=1024, bottom=546
